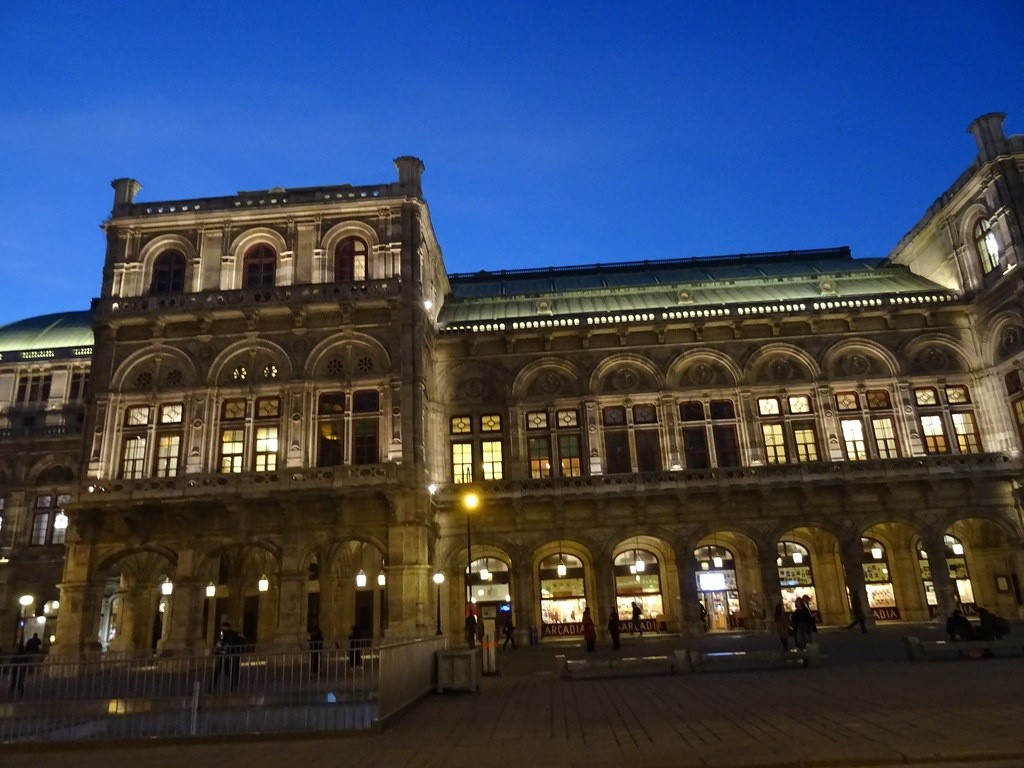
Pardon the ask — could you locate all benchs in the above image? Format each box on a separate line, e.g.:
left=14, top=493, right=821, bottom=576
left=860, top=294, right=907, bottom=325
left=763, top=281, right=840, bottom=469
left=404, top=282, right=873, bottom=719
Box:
left=554, top=642, right=822, bottom=680
left=905, top=633, right=1024, bottom=662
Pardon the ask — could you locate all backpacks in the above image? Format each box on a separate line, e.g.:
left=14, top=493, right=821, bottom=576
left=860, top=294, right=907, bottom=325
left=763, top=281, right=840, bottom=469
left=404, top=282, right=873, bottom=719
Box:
left=235, top=635, right=247, bottom=653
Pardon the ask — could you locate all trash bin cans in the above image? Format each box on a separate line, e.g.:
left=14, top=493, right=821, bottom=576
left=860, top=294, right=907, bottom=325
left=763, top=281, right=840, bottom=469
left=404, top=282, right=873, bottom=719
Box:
left=528, top=626, right=539, bottom=647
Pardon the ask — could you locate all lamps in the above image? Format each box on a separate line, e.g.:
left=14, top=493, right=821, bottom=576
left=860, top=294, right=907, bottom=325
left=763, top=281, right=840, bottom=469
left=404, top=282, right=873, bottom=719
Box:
left=951, top=523, right=963, bottom=556
left=205, top=558, right=215, bottom=597
left=161, top=561, right=173, bottom=595
left=713, top=532, right=723, bottom=568
left=792, top=529, right=802, bottom=564
left=433, top=570, right=444, bottom=635
left=377, top=556, right=386, bottom=585
left=356, top=544, right=366, bottom=586
left=629, top=537, right=644, bottom=574
left=258, top=553, right=269, bottom=591
left=872, top=526, right=882, bottom=559
left=557, top=537, right=566, bottom=578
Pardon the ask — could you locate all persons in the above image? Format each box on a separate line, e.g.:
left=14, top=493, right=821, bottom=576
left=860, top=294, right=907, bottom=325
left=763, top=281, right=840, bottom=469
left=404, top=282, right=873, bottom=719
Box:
left=947, top=607, right=993, bottom=656
left=608, top=607, right=620, bottom=650
left=349, top=625, right=367, bottom=672
left=208, top=623, right=244, bottom=691
left=583, top=608, right=597, bottom=651
left=699, top=603, right=707, bottom=632
left=465, top=613, right=476, bottom=642
left=631, top=602, right=642, bottom=635
left=775, top=596, right=818, bottom=650
left=502, top=616, right=516, bottom=648
left=847, top=591, right=867, bottom=633
left=308, top=618, right=323, bottom=675
left=10, top=633, right=42, bottom=696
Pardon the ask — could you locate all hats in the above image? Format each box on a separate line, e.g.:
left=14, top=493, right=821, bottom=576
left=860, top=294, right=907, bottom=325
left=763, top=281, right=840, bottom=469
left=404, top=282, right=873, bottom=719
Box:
left=221, top=622, right=230, bottom=627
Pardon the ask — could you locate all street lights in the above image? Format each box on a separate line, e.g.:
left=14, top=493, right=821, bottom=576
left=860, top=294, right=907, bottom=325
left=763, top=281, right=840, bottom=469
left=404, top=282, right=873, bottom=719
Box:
left=433, top=569, right=445, bottom=636
left=464, top=494, right=477, bottom=651
